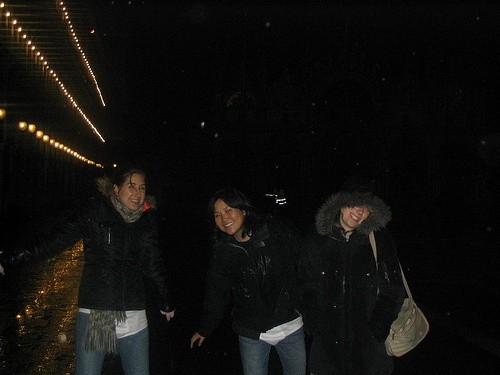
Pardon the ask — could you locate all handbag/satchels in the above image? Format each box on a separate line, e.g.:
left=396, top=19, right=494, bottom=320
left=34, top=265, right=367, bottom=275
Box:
left=384, top=298, right=429, bottom=358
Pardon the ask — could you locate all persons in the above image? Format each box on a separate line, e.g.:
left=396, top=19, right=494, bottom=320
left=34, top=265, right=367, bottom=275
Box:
left=190, top=172, right=405, bottom=375
left=0, top=166, right=176, bottom=375
